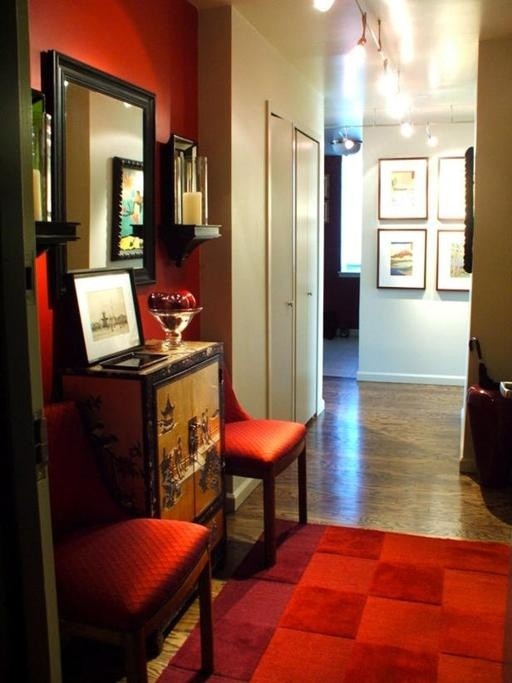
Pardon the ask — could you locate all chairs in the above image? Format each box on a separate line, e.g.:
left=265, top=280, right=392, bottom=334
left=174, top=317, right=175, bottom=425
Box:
left=220, top=372, right=308, bottom=569
left=44, top=400, right=215, bottom=683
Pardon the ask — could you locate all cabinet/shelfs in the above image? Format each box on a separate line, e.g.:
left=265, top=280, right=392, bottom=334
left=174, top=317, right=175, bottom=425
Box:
left=59, top=339, right=230, bottom=580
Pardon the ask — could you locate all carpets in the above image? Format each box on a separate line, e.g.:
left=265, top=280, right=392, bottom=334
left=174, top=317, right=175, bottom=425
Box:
left=151, top=518, right=512, bottom=683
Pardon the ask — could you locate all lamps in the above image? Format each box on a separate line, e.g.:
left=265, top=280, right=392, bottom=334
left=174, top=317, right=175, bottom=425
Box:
left=311, top=0, right=440, bottom=153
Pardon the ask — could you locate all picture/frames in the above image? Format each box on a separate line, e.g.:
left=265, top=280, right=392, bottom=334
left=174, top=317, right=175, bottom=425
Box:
left=436, top=229, right=471, bottom=292
left=377, top=157, right=429, bottom=220
left=436, top=155, right=466, bottom=221
left=68, top=266, right=146, bottom=367
left=376, top=228, right=428, bottom=290
left=110, top=155, right=145, bottom=261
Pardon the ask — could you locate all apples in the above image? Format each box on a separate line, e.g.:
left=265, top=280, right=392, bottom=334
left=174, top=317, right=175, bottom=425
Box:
left=148, top=287, right=196, bottom=309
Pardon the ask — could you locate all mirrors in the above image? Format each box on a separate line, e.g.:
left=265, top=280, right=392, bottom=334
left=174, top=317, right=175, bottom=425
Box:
left=45, top=47, right=160, bottom=289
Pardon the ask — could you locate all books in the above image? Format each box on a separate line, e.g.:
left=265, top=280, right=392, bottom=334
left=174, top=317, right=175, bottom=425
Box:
left=174, top=145, right=209, bottom=225
